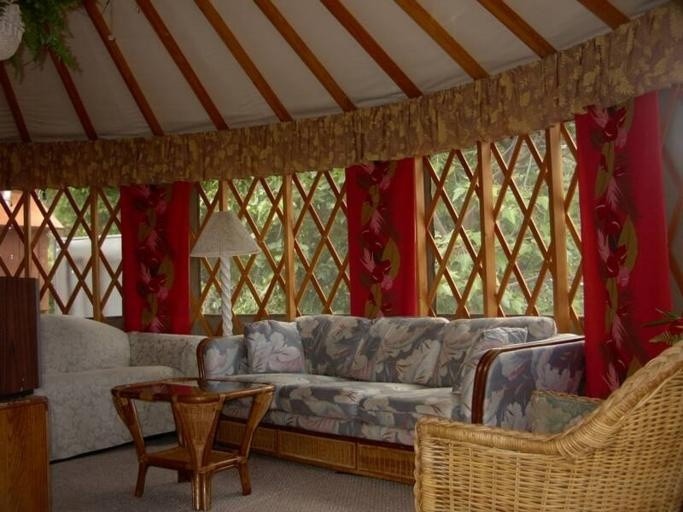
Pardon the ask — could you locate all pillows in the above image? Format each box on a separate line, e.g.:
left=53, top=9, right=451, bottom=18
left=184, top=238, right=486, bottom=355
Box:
left=524, top=385, right=601, bottom=436
left=240, top=319, right=308, bottom=376
left=452, top=326, right=530, bottom=399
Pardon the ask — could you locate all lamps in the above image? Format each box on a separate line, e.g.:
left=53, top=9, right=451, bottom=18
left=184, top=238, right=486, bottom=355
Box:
left=189, top=209, right=263, bottom=336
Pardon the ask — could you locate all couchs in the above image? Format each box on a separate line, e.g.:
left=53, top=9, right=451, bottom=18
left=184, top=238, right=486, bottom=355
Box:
left=36, top=313, right=210, bottom=465
left=196, top=310, right=585, bottom=487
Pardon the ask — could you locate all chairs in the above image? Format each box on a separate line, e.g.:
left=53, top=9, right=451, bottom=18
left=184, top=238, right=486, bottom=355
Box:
left=412, top=341, right=680, bottom=510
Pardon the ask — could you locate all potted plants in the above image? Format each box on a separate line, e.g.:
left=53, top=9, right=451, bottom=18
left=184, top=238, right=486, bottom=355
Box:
left=0, top=0, right=94, bottom=86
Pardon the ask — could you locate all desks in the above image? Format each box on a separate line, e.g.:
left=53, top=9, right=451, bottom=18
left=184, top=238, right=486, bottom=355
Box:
left=112, top=378, right=274, bottom=512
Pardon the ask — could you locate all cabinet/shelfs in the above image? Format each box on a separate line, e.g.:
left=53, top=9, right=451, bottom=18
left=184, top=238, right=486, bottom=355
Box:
left=0, top=395, right=53, bottom=512
